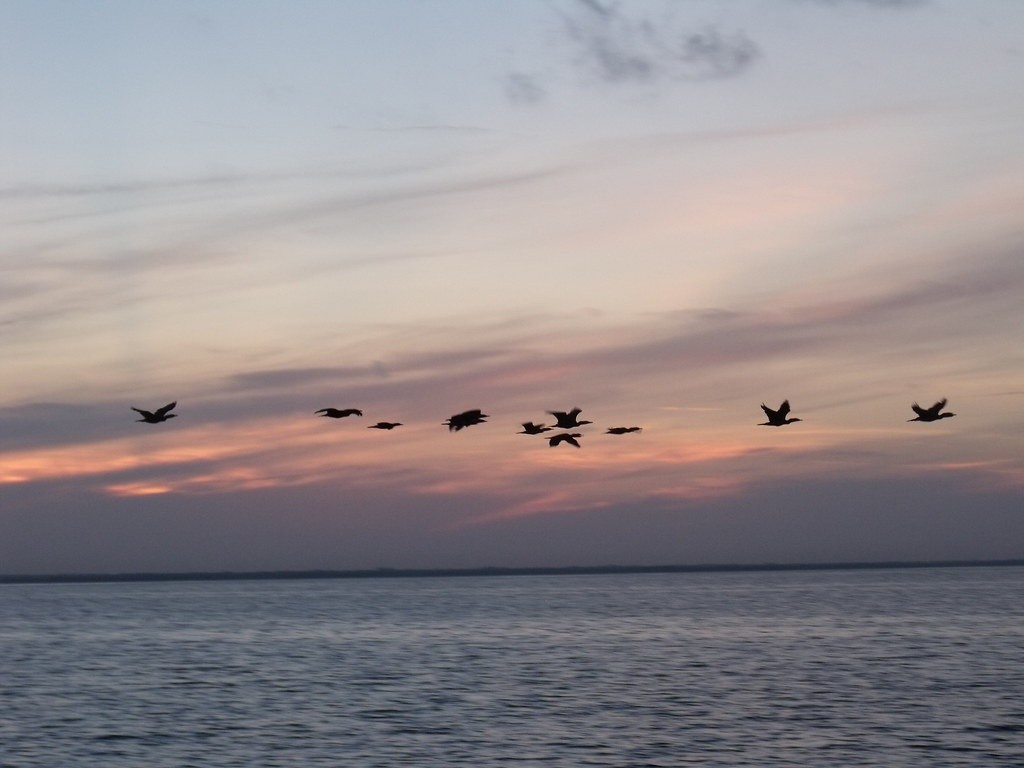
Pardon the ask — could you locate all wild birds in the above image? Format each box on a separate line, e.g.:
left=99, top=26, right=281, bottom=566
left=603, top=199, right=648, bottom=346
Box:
left=518, top=422, right=554, bottom=434
left=315, top=408, right=362, bottom=418
left=131, top=401, right=178, bottom=423
left=544, top=433, right=581, bottom=448
left=908, top=400, right=953, bottom=421
left=441, top=409, right=490, bottom=432
left=368, top=422, right=403, bottom=430
left=550, top=409, right=593, bottom=428
left=757, top=400, right=803, bottom=426
left=607, top=427, right=642, bottom=434
